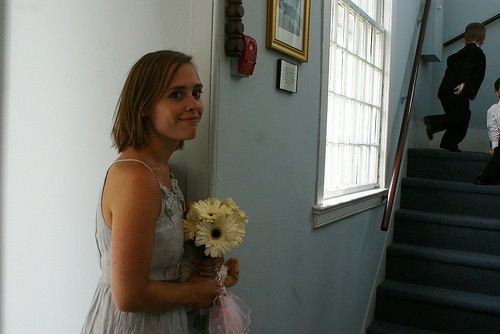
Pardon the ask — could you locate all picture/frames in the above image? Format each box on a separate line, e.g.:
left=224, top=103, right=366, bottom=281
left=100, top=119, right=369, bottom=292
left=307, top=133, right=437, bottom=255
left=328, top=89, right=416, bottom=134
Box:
left=265, top=0, right=311, bottom=64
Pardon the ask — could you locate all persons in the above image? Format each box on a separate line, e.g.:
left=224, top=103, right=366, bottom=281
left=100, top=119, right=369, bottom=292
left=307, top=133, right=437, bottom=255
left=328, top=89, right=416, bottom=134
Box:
left=423, top=22, right=487, bottom=153
left=473, top=77, right=500, bottom=186
left=80, top=50, right=227, bottom=334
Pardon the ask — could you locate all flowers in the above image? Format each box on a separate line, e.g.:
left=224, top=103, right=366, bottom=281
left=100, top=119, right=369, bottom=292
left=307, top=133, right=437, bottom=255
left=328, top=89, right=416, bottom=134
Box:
left=181, top=194, right=252, bottom=330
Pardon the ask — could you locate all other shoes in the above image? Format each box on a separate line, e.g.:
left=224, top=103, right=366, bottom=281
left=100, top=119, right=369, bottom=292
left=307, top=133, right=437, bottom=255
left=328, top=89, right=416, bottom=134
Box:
left=473, top=175, right=488, bottom=185
left=440, top=143, right=461, bottom=152
left=423, top=116, right=434, bottom=141
